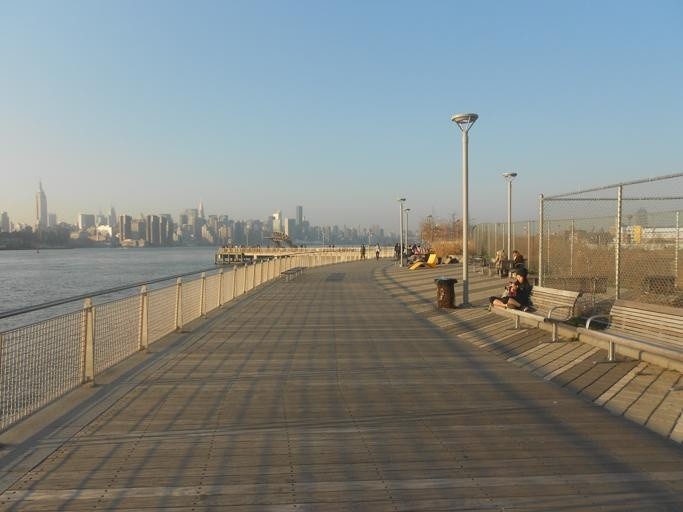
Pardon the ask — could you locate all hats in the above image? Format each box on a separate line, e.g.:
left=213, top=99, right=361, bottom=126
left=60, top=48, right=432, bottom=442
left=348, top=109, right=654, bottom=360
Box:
left=516, top=268, right=528, bottom=278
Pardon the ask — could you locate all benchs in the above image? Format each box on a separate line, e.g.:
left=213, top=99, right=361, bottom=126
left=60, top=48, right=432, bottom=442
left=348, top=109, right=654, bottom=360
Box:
left=280, top=266, right=307, bottom=281
left=489, top=285, right=682, bottom=373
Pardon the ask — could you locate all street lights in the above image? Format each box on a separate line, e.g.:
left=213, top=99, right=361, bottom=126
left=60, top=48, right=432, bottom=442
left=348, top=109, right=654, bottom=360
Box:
left=502, top=172, right=519, bottom=261
left=450, top=111, right=479, bottom=307
left=396, top=197, right=411, bottom=268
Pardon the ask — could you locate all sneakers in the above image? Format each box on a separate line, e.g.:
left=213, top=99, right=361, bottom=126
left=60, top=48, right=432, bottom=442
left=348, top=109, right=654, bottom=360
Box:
left=522, top=304, right=529, bottom=311
left=506, top=302, right=517, bottom=309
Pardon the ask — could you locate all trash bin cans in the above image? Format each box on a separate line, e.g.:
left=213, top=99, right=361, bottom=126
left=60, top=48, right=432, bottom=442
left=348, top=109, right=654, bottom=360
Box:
left=434, top=278, right=458, bottom=308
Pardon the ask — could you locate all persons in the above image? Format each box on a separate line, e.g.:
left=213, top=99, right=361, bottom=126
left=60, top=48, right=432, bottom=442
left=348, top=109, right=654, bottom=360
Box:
left=359, top=244, right=365, bottom=260
left=487, top=267, right=532, bottom=312
left=394, top=242, right=421, bottom=260
left=373, top=241, right=380, bottom=261
left=494, top=249, right=508, bottom=277
left=511, top=250, right=524, bottom=266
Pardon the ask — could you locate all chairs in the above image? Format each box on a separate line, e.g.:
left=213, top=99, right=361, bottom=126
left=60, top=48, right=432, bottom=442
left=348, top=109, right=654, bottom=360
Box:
left=409, top=253, right=437, bottom=270
